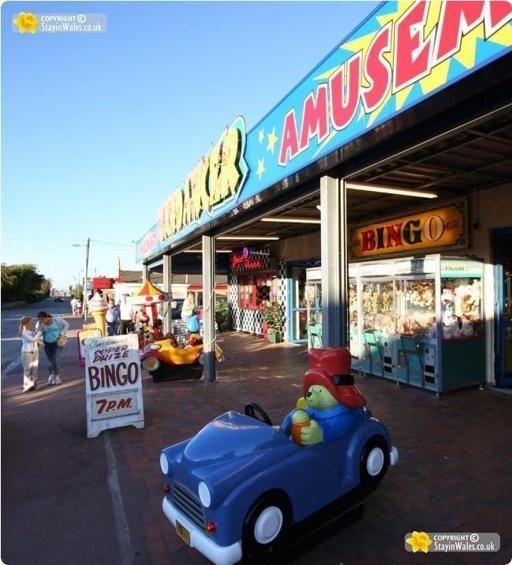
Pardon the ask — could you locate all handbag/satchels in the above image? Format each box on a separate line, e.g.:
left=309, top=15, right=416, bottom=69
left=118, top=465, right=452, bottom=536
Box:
left=57, top=334, right=68, bottom=347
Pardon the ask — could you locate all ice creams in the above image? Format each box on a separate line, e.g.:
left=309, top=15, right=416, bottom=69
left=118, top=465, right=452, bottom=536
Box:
left=88, top=292, right=109, bottom=337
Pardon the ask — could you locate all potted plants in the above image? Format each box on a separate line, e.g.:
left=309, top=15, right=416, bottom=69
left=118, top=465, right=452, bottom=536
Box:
left=215, top=298, right=233, bottom=331
left=263, top=300, right=286, bottom=343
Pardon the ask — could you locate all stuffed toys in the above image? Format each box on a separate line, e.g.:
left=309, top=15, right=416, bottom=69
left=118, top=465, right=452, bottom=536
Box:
left=439, top=278, right=481, bottom=339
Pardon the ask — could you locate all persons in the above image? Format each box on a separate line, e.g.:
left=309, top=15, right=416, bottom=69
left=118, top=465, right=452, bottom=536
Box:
left=132, top=311, right=150, bottom=331
left=181, top=291, right=195, bottom=322
left=106, top=299, right=120, bottom=336
left=70, top=295, right=80, bottom=317
left=19, top=316, right=44, bottom=392
left=36, top=311, right=70, bottom=385
left=186, top=307, right=200, bottom=335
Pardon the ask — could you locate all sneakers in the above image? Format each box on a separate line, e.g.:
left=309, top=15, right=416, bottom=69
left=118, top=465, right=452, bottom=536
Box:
left=55, top=375, right=63, bottom=384
left=48, top=375, right=54, bottom=384
